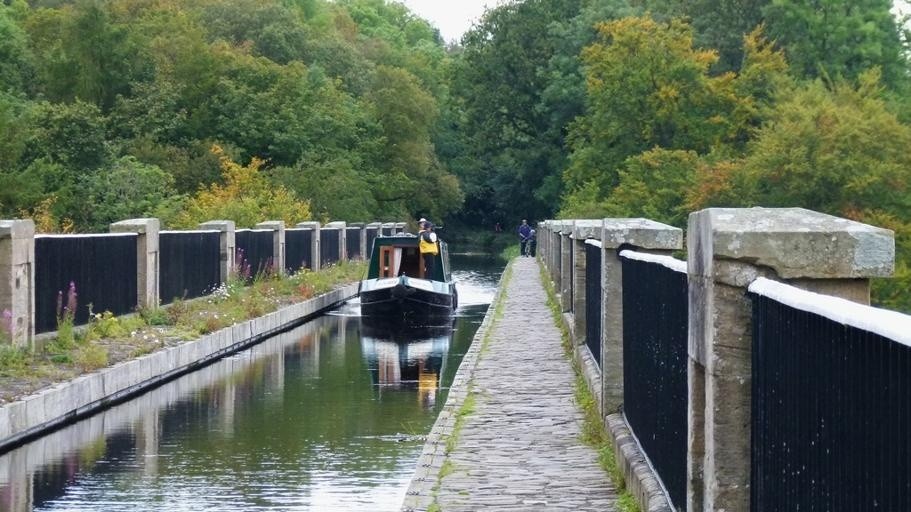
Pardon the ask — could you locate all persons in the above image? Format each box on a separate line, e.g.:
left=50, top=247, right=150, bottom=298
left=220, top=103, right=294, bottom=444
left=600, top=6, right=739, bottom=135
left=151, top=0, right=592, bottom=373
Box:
left=519, top=216, right=534, bottom=257
left=418, top=221, right=438, bottom=280
left=494, top=220, right=502, bottom=233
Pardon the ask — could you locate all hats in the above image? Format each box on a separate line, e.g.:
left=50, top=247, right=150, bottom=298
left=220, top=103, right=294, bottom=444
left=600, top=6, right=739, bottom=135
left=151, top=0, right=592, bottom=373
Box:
left=424, top=221, right=436, bottom=227
left=416, top=217, right=426, bottom=224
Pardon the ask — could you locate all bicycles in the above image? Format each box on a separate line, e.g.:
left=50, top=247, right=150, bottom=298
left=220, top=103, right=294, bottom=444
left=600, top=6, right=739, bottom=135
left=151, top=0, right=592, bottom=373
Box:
left=524, top=237, right=536, bottom=258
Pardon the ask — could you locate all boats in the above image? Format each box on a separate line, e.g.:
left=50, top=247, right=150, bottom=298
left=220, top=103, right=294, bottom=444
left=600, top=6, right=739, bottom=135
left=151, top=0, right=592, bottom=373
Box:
left=357, top=326, right=453, bottom=404
left=358, top=232, right=458, bottom=326
